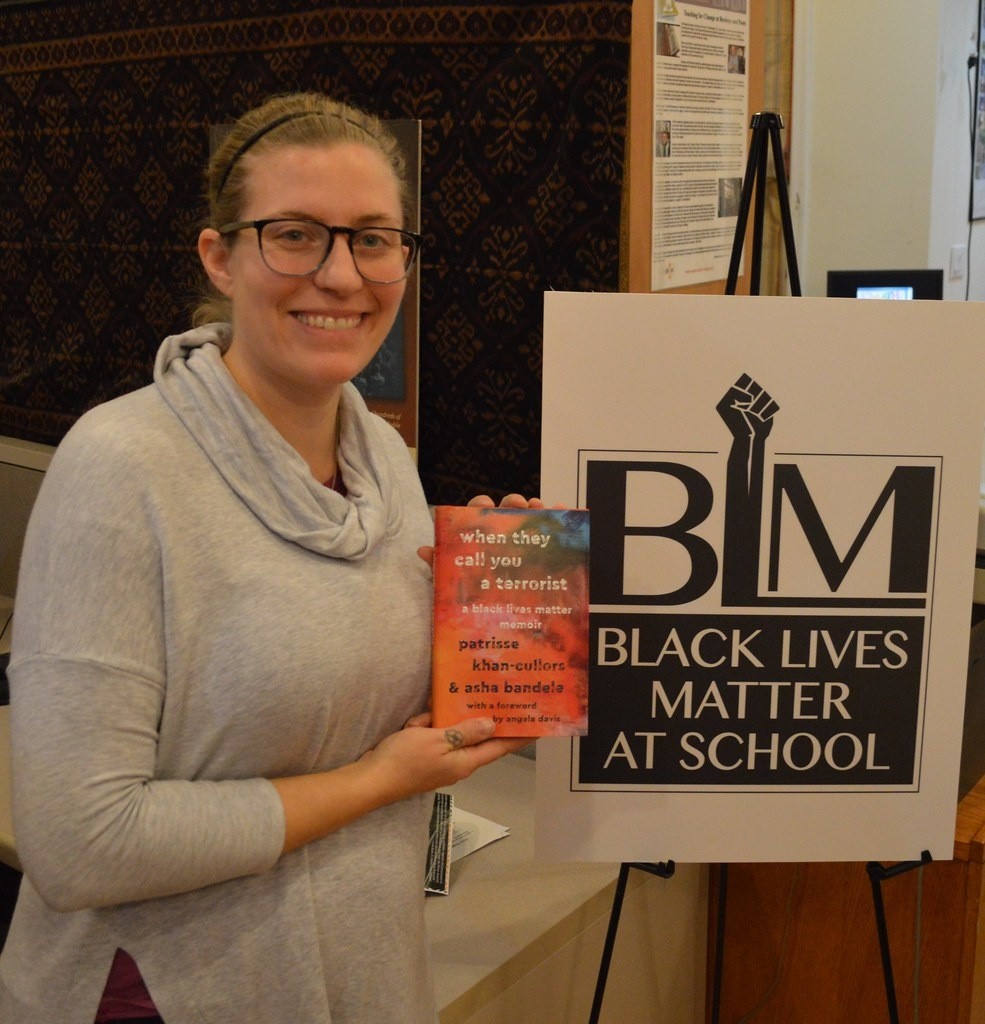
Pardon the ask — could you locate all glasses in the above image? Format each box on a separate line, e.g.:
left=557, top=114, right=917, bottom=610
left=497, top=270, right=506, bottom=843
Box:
left=217, top=217, right=426, bottom=284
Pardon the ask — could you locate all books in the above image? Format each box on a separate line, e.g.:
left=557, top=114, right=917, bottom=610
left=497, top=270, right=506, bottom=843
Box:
left=433, top=503, right=590, bottom=737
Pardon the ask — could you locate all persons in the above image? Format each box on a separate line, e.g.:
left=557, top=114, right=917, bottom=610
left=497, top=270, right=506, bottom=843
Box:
left=0, top=93, right=573, bottom=1024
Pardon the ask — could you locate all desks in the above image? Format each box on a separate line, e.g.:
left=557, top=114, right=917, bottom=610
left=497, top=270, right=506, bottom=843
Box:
left=0, top=594, right=709, bottom=1024
